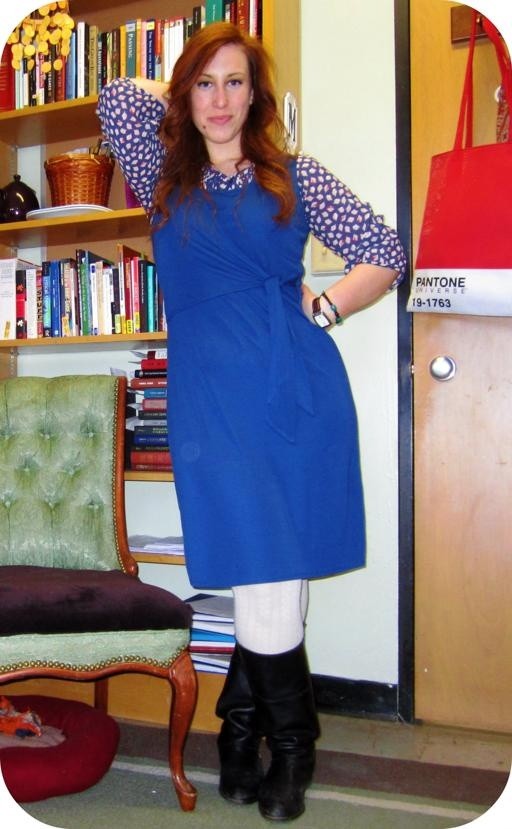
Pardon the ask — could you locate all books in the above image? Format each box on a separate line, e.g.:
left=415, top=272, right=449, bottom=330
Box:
left=182, top=593, right=240, bottom=675
left=0, top=244, right=169, bottom=340
left=123, top=347, right=175, bottom=473
left=0, top=0, right=262, bottom=111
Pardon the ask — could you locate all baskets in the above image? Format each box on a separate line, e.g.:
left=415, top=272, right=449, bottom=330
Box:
left=43, top=149, right=113, bottom=211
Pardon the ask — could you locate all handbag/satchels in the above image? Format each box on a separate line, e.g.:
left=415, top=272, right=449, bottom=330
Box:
left=407, top=140, right=512, bottom=320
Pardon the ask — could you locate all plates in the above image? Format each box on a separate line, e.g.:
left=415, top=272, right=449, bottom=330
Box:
left=26, top=204, right=114, bottom=218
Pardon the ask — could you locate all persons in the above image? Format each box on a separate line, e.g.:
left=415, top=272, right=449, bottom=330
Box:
left=96, top=22, right=406, bottom=820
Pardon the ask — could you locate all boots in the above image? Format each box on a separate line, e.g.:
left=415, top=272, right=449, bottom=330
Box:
left=215, top=645, right=321, bottom=820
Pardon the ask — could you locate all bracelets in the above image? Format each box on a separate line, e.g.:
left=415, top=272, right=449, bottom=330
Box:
left=321, top=290, right=343, bottom=326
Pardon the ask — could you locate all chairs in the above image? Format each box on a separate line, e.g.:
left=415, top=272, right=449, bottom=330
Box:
left=0, top=376, right=201, bottom=809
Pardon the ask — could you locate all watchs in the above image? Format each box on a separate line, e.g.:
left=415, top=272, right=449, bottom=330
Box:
left=311, top=296, right=332, bottom=330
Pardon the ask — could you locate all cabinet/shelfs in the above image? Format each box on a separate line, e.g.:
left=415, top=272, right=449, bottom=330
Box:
left=0, top=0, right=302, bottom=733
